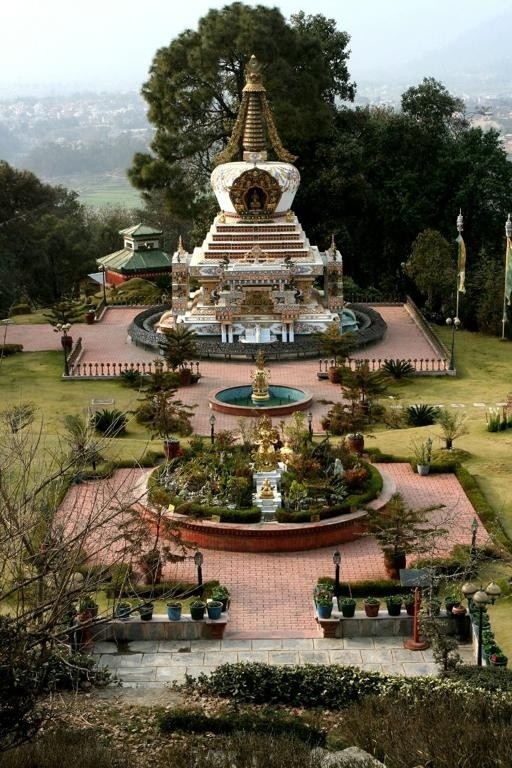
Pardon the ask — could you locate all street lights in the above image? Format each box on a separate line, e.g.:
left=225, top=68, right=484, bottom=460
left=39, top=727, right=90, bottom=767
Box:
left=334, top=548, right=342, bottom=612
left=445, top=316, right=460, bottom=369
left=57, top=323, right=71, bottom=377
left=194, top=551, right=205, bottom=601
left=209, top=414, right=216, bottom=445
left=470, top=518, right=479, bottom=554
left=89, top=440, right=96, bottom=471
left=462, top=577, right=500, bottom=666
left=426, top=438, right=433, bottom=463
left=97, top=264, right=108, bottom=306
left=308, top=414, right=313, bottom=441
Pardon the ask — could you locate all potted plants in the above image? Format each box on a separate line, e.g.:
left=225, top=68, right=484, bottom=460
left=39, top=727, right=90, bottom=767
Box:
left=320, top=401, right=377, bottom=457
left=404, top=435, right=436, bottom=476
left=77, top=585, right=231, bottom=621
left=311, top=322, right=361, bottom=383
left=133, top=390, right=199, bottom=461
left=339, top=361, right=396, bottom=415
left=108, top=506, right=199, bottom=585
left=469, top=603, right=508, bottom=667
left=351, top=491, right=450, bottom=579
left=427, top=409, right=470, bottom=449
left=157, top=322, right=201, bottom=384
left=312, top=583, right=467, bottom=619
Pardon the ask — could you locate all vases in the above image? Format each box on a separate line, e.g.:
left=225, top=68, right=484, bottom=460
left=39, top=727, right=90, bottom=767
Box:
left=85, top=312, right=95, bottom=325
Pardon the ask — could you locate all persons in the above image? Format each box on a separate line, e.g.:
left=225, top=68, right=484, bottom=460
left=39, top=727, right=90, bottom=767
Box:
left=253, top=413, right=280, bottom=471
